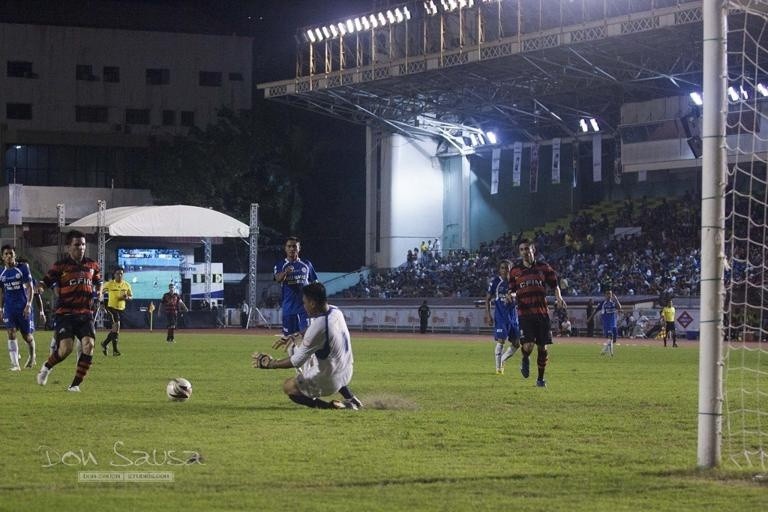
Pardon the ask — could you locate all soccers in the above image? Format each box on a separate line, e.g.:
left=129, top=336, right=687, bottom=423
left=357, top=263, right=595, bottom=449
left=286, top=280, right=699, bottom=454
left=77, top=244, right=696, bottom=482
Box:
left=168, top=379, right=191, bottom=401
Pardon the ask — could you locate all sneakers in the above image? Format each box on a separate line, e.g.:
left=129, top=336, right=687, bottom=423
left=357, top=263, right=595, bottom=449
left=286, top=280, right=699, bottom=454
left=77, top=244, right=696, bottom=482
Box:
left=67, top=385, right=81, bottom=392
left=24, top=359, right=37, bottom=368
left=536, top=378, right=545, bottom=387
left=330, top=396, right=363, bottom=410
left=520, top=358, right=530, bottom=377
left=100, top=342, right=108, bottom=356
left=113, top=350, right=120, bottom=356
left=8, top=366, right=21, bottom=371
left=497, top=361, right=503, bottom=374
left=37, top=361, right=52, bottom=385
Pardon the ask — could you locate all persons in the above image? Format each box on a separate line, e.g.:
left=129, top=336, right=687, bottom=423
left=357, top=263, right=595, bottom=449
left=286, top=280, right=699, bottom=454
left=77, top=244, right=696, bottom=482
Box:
left=275, top=237, right=319, bottom=357
left=418, top=300, right=431, bottom=334
left=200, top=300, right=249, bottom=328
left=553, top=290, right=678, bottom=356
left=253, top=283, right=363, bottom=410
left=485, top=237, right=567, bottom=386
left=158, top=284, right=189, bottom=343
left=536, top=187, right=767, bottom=339
left=342, top=229, right=532, bottom=298
left=0, top=229, right=133, bottom=391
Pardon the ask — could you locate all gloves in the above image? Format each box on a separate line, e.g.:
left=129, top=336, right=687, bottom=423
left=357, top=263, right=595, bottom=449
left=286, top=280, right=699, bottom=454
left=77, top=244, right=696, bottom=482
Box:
left=271, top=335, right=295, bottom=353
left=250, top=351, right=273, bottom=370
